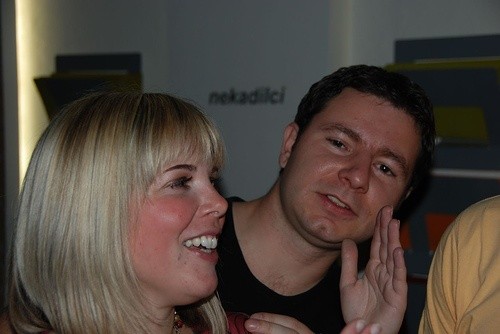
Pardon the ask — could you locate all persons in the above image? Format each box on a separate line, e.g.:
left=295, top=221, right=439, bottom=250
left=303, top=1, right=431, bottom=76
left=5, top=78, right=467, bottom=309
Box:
left=0, top=89, right=320, bottom=334
left=417, top=194, right=499, bottom=334
left=202, top=62, right=438, bottom=334
